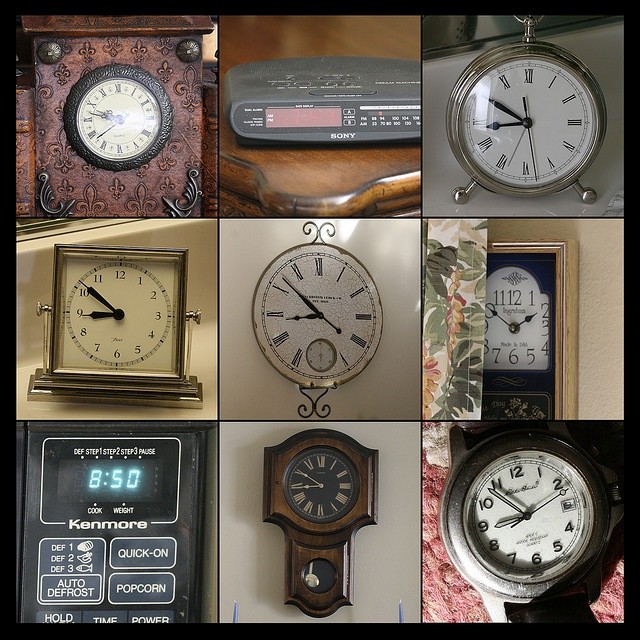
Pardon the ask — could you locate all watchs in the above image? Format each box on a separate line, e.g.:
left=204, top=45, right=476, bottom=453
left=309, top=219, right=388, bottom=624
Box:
left=437, top=422, right=624, bottom=623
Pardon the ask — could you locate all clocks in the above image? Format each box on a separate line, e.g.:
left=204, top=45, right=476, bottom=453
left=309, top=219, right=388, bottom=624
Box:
left=253, top=221, right=384, bottom=419
left=485, top=239, right=579, bottom=420
left=447, top=16, right=606, bottom=206
left=28, top=241, right=204, bottom=413
left=261, top=428, right=380, bottom=618
left=62, top=62, right=172, bottom=173
